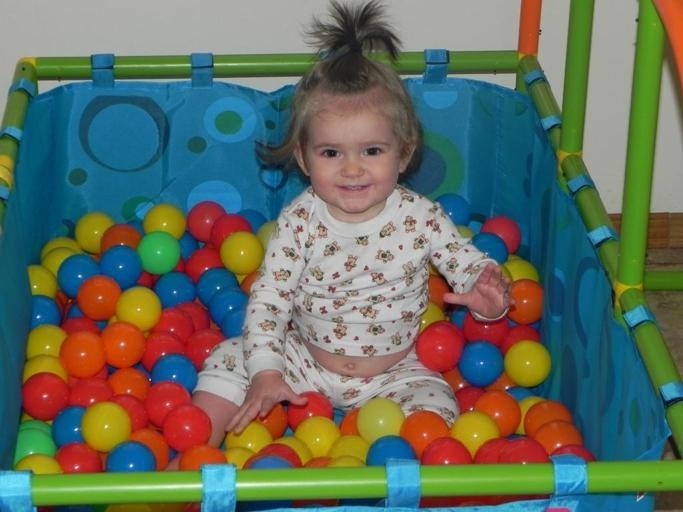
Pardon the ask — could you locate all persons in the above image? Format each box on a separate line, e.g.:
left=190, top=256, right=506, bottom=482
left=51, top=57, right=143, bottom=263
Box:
left=163, top=0, right=516, bottom=480
